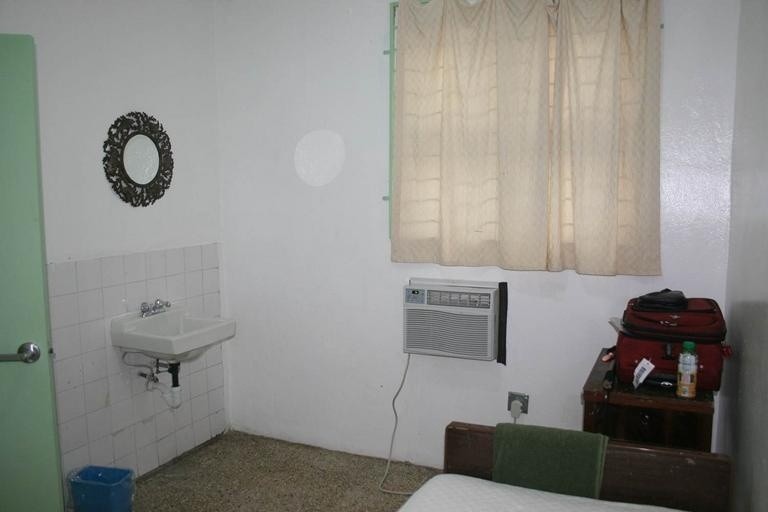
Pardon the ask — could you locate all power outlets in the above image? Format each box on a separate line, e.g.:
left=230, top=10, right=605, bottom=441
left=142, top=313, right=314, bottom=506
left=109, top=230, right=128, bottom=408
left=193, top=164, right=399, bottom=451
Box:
left=507, top=392, right=529, bottom=415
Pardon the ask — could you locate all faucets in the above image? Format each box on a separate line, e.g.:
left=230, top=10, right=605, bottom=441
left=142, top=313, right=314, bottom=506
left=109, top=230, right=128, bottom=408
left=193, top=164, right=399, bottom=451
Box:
left=139, top=299, right=171, bottom=319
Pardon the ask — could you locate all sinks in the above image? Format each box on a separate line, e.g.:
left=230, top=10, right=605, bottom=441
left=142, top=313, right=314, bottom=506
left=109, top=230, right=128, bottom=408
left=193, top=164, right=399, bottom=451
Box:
left=110, top=304, right=237, bottom=363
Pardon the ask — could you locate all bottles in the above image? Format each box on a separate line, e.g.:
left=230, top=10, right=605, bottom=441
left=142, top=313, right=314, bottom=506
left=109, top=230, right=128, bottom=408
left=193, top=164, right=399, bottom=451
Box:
left=675, top=341, right=699, bottom=399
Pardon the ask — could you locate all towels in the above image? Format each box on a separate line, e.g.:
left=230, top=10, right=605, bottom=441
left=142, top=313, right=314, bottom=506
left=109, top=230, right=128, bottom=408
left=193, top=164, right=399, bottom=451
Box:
left=491, top=424, right=608, bottom=500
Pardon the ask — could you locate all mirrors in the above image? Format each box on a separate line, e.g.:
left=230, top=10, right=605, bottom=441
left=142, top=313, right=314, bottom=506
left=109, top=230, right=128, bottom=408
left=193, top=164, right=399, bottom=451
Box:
left=105, top=113, right=174, bottom=206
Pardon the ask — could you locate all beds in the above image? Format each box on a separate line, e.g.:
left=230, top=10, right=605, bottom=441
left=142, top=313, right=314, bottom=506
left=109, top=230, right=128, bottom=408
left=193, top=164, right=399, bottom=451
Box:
left=397, top=420, right=735, bottom=512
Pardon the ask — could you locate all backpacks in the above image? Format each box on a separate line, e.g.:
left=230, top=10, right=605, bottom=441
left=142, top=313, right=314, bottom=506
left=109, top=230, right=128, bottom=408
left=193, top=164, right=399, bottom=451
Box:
left=608, top=289, right=727, bottom=342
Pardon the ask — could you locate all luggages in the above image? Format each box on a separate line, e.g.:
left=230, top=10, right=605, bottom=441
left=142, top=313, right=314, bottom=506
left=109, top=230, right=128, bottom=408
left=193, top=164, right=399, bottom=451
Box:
left=602, top=333, right=723, bottom=390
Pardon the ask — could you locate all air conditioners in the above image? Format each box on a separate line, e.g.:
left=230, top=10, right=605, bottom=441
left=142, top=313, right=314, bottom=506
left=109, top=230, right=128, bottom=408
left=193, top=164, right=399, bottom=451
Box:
left=402, top=283, right=499, bottom=361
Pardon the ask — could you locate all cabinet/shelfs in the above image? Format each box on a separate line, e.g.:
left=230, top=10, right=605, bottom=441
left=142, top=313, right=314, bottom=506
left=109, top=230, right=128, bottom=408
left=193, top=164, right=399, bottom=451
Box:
left=582, top=347, right=714, bottom=451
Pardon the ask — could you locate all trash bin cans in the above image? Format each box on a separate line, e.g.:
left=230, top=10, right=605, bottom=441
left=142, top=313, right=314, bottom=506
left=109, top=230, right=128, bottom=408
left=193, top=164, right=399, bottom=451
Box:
left=68, top=466, right=132, bottom=512
